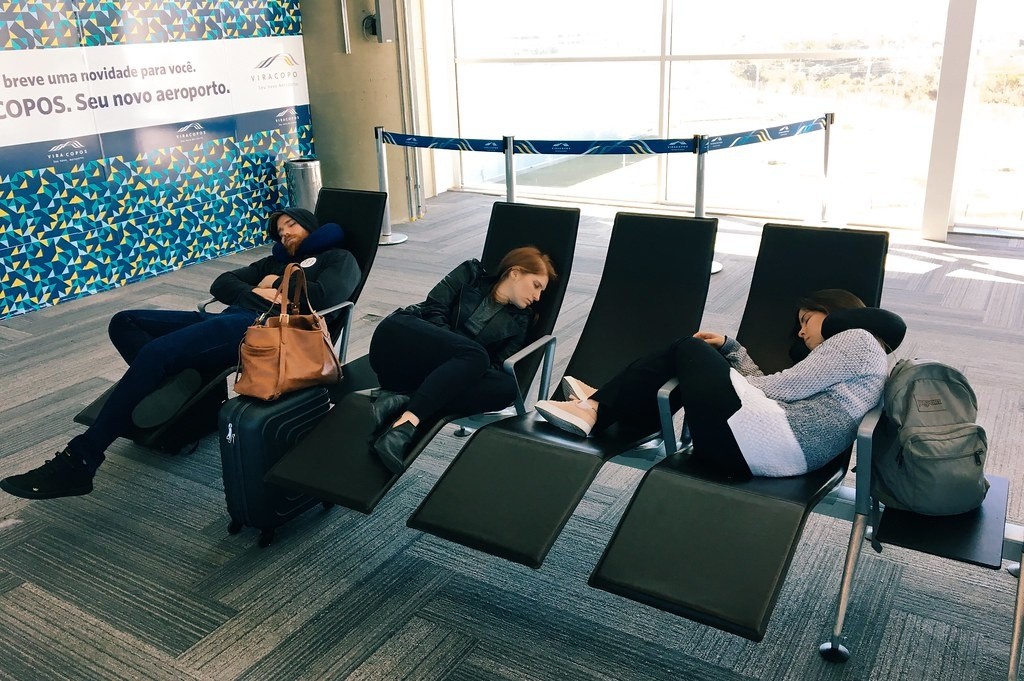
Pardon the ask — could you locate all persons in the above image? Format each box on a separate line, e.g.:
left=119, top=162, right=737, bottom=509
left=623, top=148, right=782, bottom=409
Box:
left=367, top=246, right=557, bottom=476
left=0, top=208, right=361, bottom=501
left=534, top=286, right=908, bottom=484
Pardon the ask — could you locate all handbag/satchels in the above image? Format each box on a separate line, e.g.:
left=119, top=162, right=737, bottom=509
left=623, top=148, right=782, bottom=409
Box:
left=232, top=262, right=344, bottom=401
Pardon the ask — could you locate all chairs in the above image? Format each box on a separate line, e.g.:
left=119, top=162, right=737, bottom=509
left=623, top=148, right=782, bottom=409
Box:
left=406, top=208, right=719, bottom=570
left=263, top=202, right=581, bottom=516
left=587, top=222, right=889, bottom=643
left=72, top=188, right=387, bottom=447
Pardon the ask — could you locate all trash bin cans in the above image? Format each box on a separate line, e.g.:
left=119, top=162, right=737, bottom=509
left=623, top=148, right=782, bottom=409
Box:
left=282, top=157, right=324, bottom=215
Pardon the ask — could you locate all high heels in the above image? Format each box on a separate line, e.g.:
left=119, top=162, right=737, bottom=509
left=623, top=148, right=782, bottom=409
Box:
left=370, top=388, right=413, bottom=424
left=368, top=413, right=418, bottom=475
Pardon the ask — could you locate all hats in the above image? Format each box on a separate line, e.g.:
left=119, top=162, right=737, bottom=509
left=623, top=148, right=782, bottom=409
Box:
left=269, top=211, right=283, bottom=244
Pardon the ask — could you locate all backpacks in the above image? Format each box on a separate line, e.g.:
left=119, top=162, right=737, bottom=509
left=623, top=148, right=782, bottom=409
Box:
left=869, top=358, right=990, bottom=551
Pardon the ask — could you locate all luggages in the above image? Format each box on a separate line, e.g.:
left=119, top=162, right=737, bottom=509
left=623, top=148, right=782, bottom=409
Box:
left=217, top=385, right=332, bottom=547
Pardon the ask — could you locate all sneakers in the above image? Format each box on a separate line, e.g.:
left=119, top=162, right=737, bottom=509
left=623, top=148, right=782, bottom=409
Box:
left=535, top=398, right=599, bottom=436
left=562, top=376, right=598, bottom=402
left=0, top=433, right=106, bottom=499
left=133, top=367, right=202, bottom=429
